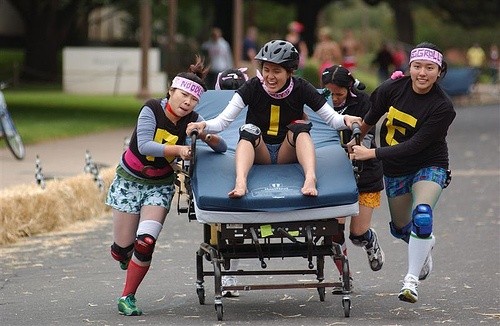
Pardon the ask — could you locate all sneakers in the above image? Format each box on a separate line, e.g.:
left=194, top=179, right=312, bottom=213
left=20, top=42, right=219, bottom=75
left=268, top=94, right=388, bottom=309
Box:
left=331, top=275, right=353, bottom=294
left=366, top=228, right=385, bottom=271
left=120, top=260, right=133, bottom=271
left=117, top=294, right=142, bottom=316
left=398, top=275, right=421, bottom=304
left=222, top=277, right=239, bottom=297
left=417, top=235, right=436, bottom=280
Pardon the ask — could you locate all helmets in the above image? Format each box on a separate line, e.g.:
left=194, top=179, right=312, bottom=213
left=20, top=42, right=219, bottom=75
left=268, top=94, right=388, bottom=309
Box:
left=252, top=40, right=300, bottom=70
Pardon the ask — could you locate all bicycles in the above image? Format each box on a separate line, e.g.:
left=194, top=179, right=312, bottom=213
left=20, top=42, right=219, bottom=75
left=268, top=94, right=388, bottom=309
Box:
left=0, top=76, right=26, bottom=162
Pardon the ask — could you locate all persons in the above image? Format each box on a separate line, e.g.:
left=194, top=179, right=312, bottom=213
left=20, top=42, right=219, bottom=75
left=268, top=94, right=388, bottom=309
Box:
left=345, top=42, right=457, bottom=303
left=214, top=68, right=248, bottom=297
left=320, top=65, right=384, bottom=295
left=190, top=20, right=500, bottom=95
left=186, top=40, right=363, bottom=197
left=104, top=55, right=227, bottom=316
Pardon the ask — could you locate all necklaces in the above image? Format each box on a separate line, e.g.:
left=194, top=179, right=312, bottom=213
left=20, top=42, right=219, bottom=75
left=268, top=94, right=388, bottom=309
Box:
left=166, top=103, right=181, bottom=119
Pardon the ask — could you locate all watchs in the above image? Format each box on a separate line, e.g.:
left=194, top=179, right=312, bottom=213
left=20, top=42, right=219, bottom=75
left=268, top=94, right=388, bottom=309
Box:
left=203, top=133, right=212, bottom=142
